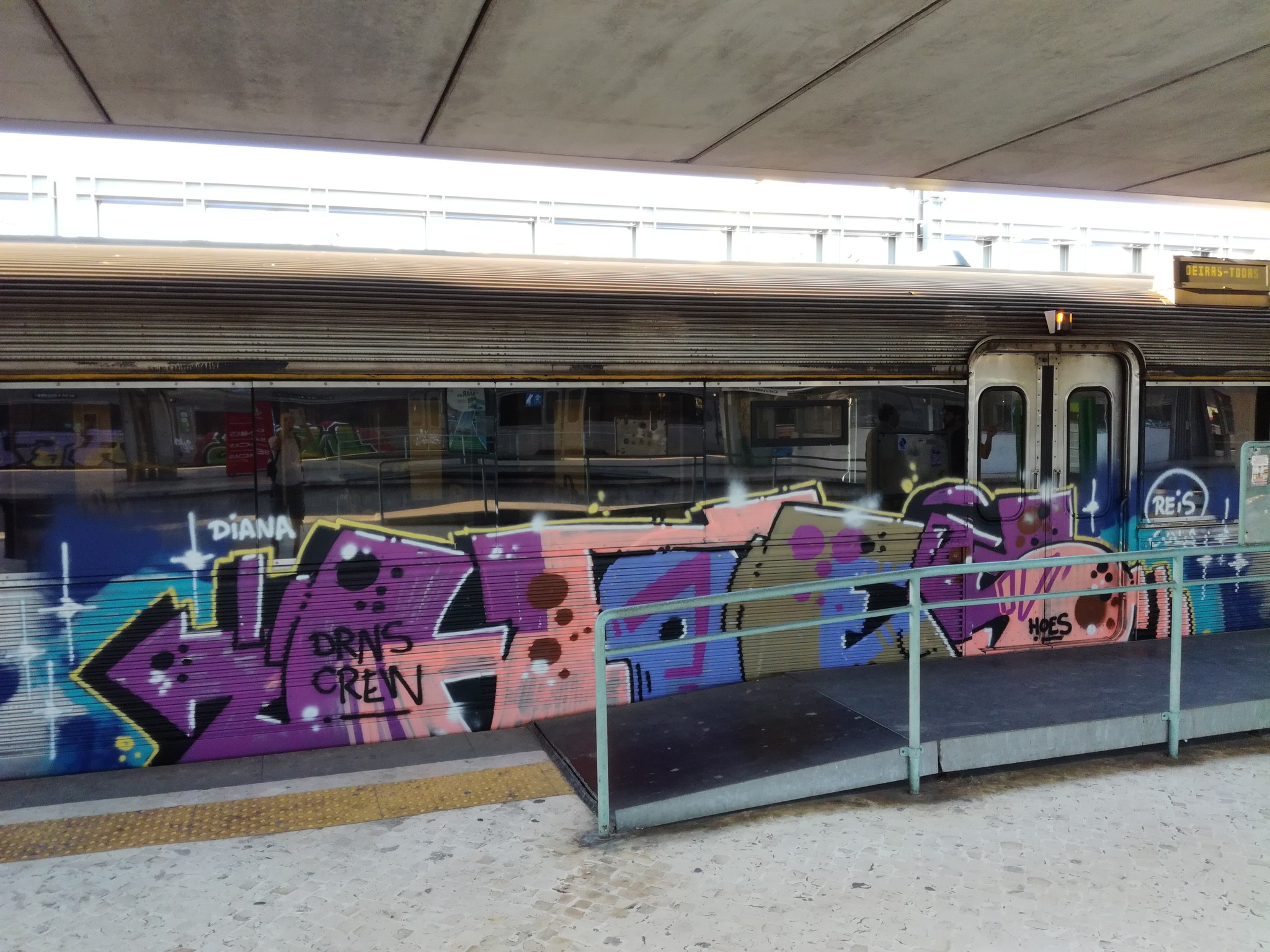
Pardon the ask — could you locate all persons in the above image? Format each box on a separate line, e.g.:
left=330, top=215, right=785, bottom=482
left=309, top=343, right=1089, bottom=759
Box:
left=265, top=411, right=304, bottom=549
left=981, top=428, right=996, bottom=459
left=866, top=403, right=909, bottom=515
